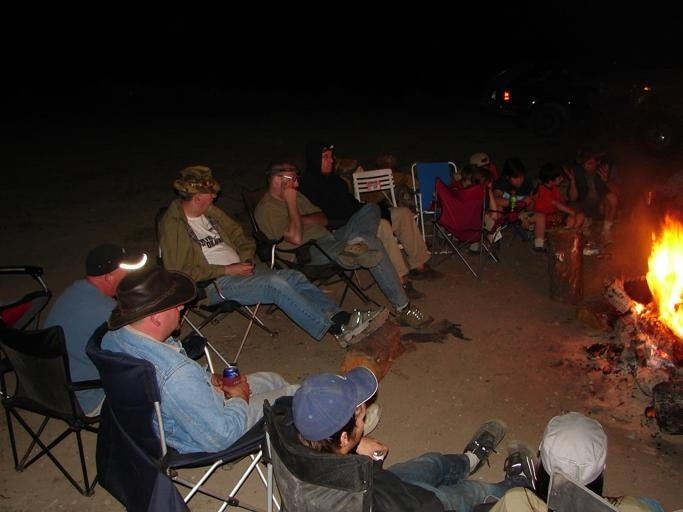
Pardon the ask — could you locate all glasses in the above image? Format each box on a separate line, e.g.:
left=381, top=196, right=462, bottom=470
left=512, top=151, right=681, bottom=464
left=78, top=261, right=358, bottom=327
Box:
left=277, top=174, right=297, bottom=182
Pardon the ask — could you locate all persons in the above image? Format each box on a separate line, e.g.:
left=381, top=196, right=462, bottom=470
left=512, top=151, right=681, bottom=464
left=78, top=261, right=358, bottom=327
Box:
left=561, top=155, right=617, bottom=233
left=157, top=166, right=390, bottom=348
left=510, top=163, right=533, bottom=197
left=273, top=365, right=538, bottom=512
left=101, top=268, right=381, bottom=454
left=492, top=412, right=664, bottom=512
left=524, top=162, right=578, bottom=250
left=254, top=162, right=435, bottom=328
left=308, top=139, right=446, bottom=301
left=449, top=153, right=512, bottom=257
left=43, top=244, right=170, bottom=418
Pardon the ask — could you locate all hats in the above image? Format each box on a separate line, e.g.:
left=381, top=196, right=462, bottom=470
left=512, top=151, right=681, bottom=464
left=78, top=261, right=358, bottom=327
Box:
left=538, top=411, right=608, bottom=486
left=291, top=366, right=379, bottom=442
left=86, top=243, right=143, bottom=276
left=173, top=165, right=221, bottom=194
left=107, top=267, right=197, bottom=330
left=469, top=152, right=490, bottom=168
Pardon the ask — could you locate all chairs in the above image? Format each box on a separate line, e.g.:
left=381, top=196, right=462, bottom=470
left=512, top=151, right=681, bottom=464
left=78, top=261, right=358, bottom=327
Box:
left=263, top=399, right=372, bottom=512
left=352, top=168, right=398, bottom=211
left=86, top=321, right=273, bottom=512
left=240, top=186, right=370, bottom=317
left=154, top=216, right=278, bottom=343
left=435, top=179, right=500, bottom=280
left=1, top=318, right=108, bottom=497
left=0, top=264, right=52, bottom=404
left=412, top=161, right=460, bottom=255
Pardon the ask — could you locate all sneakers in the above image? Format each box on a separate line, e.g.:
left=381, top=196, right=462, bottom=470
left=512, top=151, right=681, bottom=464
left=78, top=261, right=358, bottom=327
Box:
left=414, top=263, right=443, bottom=280
left=463, top=419, right=507, bottom=476
left=335, top=240, right=384, bottom=268
left=503, top=440, right=538, bottom=490
left=403, top=281, right=427, bottom=300
left=397, top=303, right=436, bottom=330
left=340, top=303, right=390, bottom=345
left=334, top=333, right=348, bottom=348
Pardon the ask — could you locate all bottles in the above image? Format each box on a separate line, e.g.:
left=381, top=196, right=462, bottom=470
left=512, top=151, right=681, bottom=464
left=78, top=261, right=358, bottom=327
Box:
left=222, top=367, right=240, bottom=400
left=373, top=451, right=384, bottom=469
left=508, top=190, right=517, bottom=221
